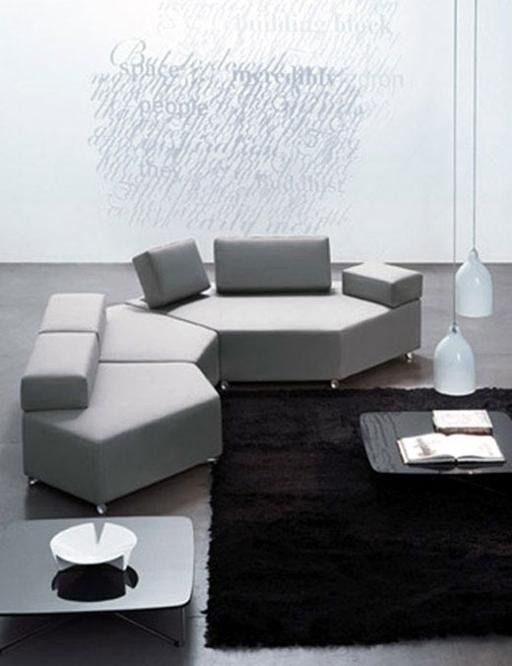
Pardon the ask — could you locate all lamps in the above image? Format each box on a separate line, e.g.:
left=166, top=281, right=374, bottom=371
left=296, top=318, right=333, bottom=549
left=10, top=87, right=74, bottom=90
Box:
left=433, top=0, right=477, bottom=398
left=455, top=0, right=493, bottom=319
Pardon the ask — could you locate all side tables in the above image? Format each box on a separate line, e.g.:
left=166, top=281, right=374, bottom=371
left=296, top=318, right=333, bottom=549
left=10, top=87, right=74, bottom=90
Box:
left=0, top=516, right=195, bottom=656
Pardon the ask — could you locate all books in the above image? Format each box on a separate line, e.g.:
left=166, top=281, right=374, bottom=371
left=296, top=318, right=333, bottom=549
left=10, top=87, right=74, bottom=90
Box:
left=395, top=432, right=508, bottom=465
left=431, top=408, right=494, bottom=435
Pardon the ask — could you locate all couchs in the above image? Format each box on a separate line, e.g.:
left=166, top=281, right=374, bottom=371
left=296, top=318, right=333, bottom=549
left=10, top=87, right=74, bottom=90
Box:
left=20, top=234, right=423, bottom=516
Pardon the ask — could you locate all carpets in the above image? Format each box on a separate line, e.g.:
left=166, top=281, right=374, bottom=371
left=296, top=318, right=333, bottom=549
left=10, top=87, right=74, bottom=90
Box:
left=201, top=387, right=512, bottom=648
